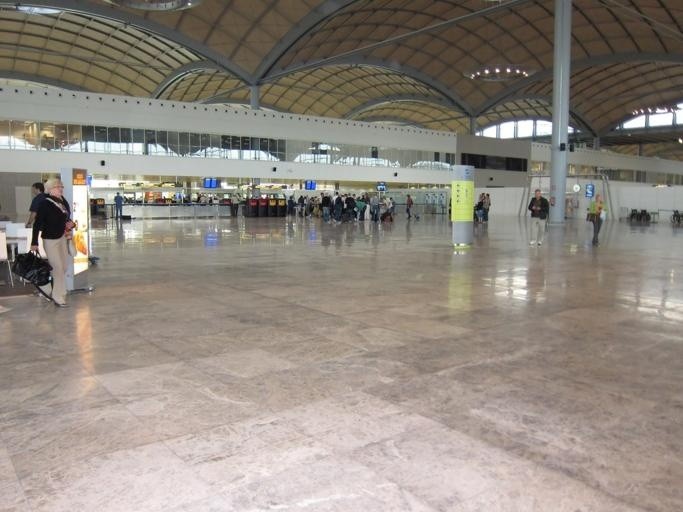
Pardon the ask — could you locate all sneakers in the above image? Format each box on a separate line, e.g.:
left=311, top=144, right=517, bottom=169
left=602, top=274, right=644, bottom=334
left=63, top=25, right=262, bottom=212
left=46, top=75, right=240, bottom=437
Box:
left=529, top=240, right=543, bottom=245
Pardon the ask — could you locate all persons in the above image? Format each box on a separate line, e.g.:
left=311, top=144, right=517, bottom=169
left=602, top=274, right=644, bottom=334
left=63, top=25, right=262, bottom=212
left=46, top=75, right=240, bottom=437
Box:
left=528, top=190, right=549, bottom=246
left=199, top=195, right=206, bottom=206
left=287, top=192, right=395, bottom=223
left=114, top=192, right=123, bottom=220
left=124, top=196, right=128, bottom=204
left=590, top=194, right=608, bottom=247
left=406, top=194, right=414, bottom=218
left=230, top=195, right=239, bottom=217
left=26, top=177, right=76, bottom=307
left=479, top=193, right=491, bottom=221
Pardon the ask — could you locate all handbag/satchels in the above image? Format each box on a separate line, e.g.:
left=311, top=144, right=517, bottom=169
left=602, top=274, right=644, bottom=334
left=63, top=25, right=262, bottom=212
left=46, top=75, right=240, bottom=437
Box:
left=11, top=249, right=52, bottom=286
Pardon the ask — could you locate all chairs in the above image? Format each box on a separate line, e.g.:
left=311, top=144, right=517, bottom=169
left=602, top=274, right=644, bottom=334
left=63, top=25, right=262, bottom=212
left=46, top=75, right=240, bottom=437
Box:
left=0, top=220, right=48, bottom=288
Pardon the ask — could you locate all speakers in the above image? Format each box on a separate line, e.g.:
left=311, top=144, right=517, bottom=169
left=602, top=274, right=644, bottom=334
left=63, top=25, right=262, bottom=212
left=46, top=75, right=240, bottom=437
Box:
left=490, top=177, right=492, bottom=181
left=570, top=144, right=574, bottom=152
left=272, top=167, right=276, bottom=172
left=560, top=143, right=565, bottom=151
left=394, top=172, right=398, bottom=177
left=101, top=159, right=105, bottom=166
